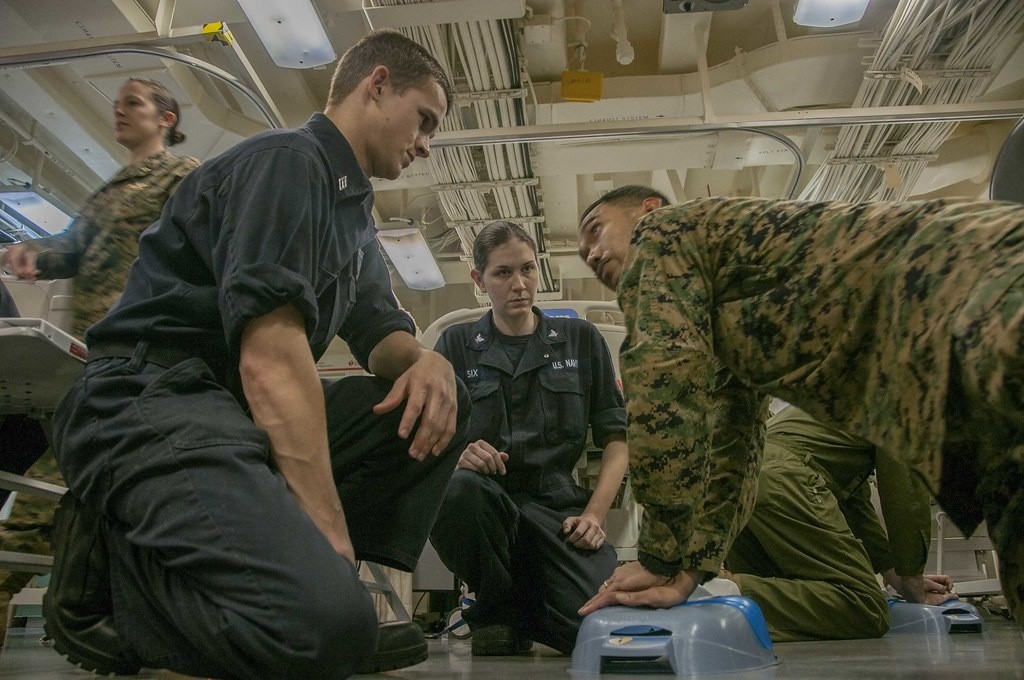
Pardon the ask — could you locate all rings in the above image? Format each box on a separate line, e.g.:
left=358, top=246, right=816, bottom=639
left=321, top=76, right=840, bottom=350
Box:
left=603, top=582, right=608, bottom=588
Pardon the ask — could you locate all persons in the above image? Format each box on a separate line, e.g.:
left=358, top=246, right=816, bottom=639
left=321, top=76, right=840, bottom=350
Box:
left=41, top=29, right=471, bottom=680
left=577, top=185, right=1024, bottom=626
left=428, top=221, right=627, bottom=657
left=0, top=77, right=203, bottom=651
left=717, top=404, right=959, bottom=643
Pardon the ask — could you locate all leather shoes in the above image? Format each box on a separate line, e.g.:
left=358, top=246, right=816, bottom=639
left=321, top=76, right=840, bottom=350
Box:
left=374, top=620, right=429, bottom=672
left=471, top=623, right=533, bottom=656
left=43, top=490, right=135, bottom=676
